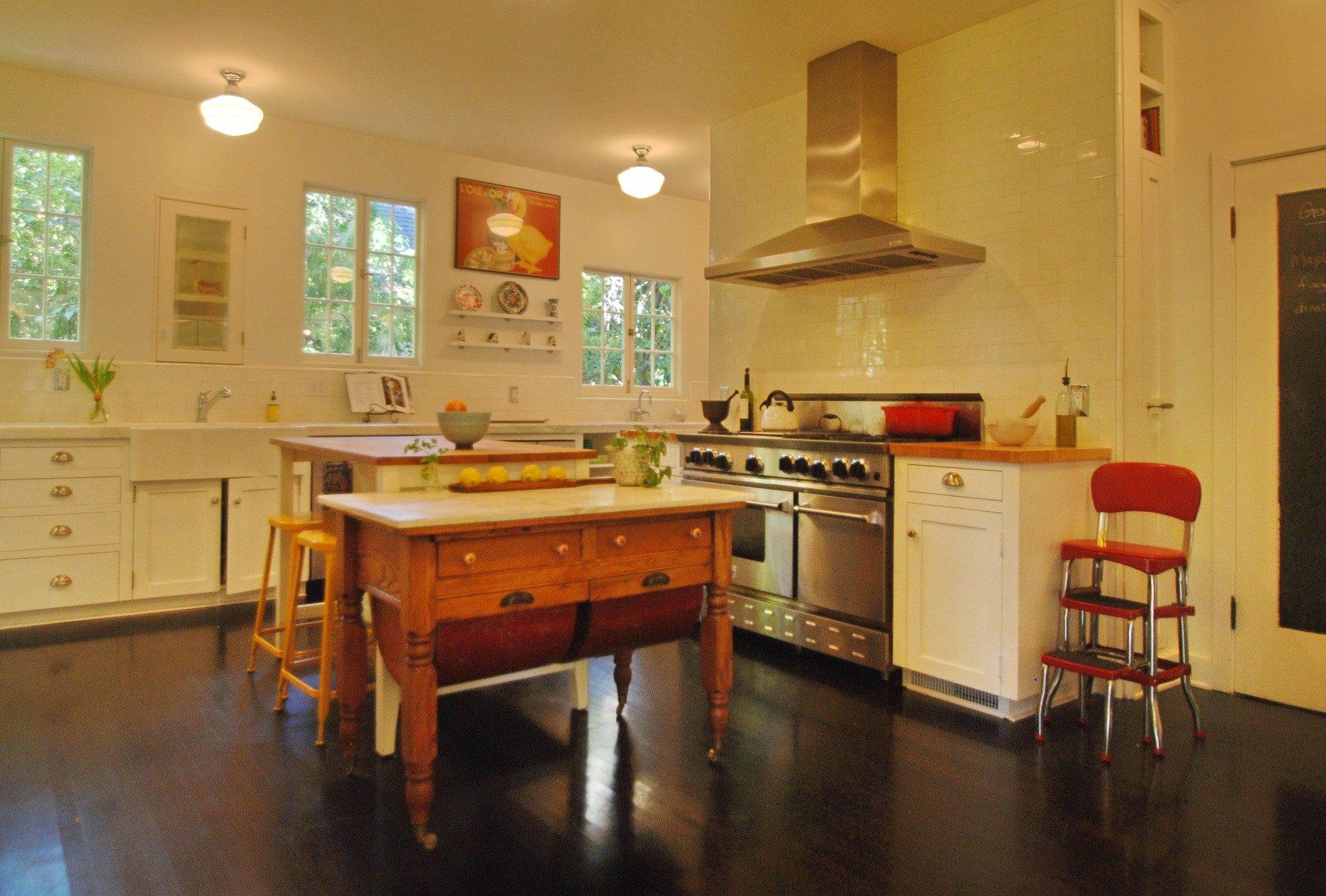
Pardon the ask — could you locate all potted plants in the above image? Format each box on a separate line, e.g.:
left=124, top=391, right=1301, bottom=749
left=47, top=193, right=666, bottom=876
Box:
left=604, top=422, right=675, bottom=488
left=65, top=350, right=121, bottom=423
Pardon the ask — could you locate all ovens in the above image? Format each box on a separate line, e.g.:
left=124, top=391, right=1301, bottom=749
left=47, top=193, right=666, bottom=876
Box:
left=680, top=477, right=892, bottom=672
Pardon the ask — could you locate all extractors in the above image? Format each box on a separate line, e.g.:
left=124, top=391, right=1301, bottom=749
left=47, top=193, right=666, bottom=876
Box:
left=705, top=43, right=985, bottom=290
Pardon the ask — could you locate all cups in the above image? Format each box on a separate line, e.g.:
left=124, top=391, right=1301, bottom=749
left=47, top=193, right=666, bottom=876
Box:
left=549, top=298, right=560, bottom=317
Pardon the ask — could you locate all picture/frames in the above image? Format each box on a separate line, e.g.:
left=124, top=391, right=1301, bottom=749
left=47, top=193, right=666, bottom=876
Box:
left=454, top=177, right=560, bottom=281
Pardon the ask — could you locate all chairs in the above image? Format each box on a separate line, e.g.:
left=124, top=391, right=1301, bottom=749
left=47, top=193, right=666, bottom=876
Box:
left=1034, top=461, right=1204, bottom=766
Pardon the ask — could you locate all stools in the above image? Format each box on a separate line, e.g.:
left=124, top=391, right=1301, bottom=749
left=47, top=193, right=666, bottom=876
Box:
left=243, top=508, right=335, bottom=701
left=274, top=527, right=375, bottom=746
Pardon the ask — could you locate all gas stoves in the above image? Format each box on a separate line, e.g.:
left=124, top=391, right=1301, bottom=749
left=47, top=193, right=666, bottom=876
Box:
left=676, top=392, right=984, bottom=490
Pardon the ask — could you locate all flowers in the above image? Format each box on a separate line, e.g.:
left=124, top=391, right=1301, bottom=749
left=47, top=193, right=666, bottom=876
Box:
left=43, top=345, right=69, bottom=371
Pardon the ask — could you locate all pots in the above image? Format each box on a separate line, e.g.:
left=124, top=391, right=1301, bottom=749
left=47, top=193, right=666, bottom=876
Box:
left=880, top=402, right=961, bottom=439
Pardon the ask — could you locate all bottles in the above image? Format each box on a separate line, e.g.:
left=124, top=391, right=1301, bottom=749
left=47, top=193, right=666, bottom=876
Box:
left=1055, top=358, right=1077, bottom=448
left=740, top=368, right=755, bottom=432
left=267, top=391, right=281, bottom=422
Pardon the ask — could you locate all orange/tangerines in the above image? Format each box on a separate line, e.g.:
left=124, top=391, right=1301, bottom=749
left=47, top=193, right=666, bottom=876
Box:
left=446, top=400, right=467, bottom=411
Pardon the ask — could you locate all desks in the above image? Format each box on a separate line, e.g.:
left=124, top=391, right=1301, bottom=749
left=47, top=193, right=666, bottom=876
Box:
left=319, top=479, right=747, bottom=859
left=267, top=434, right=597, bottom=756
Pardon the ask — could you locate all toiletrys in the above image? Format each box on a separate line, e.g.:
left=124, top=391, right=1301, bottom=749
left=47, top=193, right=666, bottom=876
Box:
left=266, top=391, right=280, bottom=423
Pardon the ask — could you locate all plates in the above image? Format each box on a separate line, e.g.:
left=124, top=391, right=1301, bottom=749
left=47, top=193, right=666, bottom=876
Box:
left=455, top=284, right=483, bottom=312
left=497, top=281, right=529, bottom=315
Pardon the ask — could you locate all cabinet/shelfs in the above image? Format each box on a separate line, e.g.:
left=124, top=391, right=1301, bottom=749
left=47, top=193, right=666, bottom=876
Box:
left=126, top=475, right=300, bottom=636
left=447, top=309, right=565, bottom=354
left=892, top=454, right=1117, bottom=726
left=0, top=439, right=128, bottom=651
left=175, top=245, right=229, bottom=351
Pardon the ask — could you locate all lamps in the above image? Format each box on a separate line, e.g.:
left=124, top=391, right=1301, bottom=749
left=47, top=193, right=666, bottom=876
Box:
left=616, top=144, right=665, bottom=200
left=485, top=195, right=524, bottom=237
left=199, top=68, right=264, bottom=137
left=331, top=266, right=355, bottom=284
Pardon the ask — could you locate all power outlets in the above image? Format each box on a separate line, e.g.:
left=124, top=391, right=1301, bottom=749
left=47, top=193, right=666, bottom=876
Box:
left=1068, top=384, right=1090, bottom=417
left=508, top=385, right=519, bottom=404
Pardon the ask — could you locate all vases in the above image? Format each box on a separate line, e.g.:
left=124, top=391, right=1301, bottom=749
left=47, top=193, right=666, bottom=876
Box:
left=54, top=368, right=71, bottom=391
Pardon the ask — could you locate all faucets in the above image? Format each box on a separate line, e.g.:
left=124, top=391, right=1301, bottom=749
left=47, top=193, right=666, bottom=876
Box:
left=630, top=389, right=652, bottom=423
left=197, top=386, right=232, bottom=422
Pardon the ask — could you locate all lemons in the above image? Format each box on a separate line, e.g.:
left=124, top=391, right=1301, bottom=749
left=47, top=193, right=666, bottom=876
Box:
left=487, top=466, right=509, bottom=484
left=459, top=467, right=480, bottom=486
left=547, top=466, right=566, bottom=480
left=521, top=465, right=542, bottom=482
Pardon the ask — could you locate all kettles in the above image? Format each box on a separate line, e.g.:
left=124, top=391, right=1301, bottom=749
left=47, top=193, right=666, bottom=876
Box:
left=759, top=390, right=799, bottom=431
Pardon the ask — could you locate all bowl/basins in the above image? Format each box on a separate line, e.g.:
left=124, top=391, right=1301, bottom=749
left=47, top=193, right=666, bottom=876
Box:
left=436, top=410, right=491, bottom=450
left=984, top=418, right=1039, bottom=446
